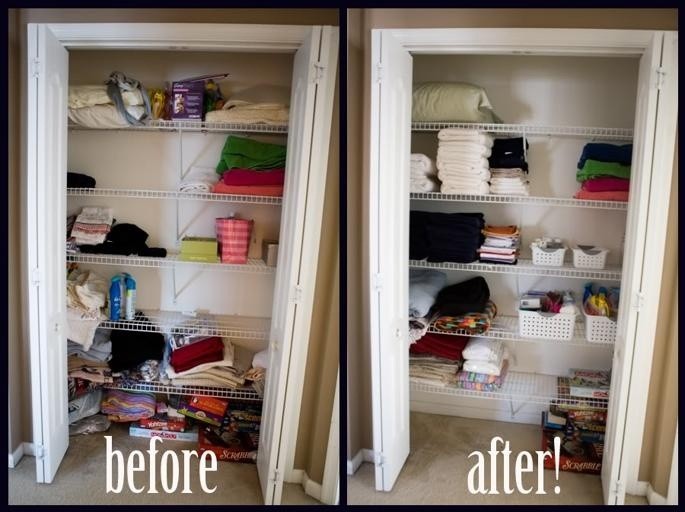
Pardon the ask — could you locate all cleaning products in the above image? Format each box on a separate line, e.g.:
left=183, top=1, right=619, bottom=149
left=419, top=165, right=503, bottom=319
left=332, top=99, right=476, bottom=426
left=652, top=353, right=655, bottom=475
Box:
left=109, top=277, right=122, bottom=320
left=126, top=276, right=136, bottom=321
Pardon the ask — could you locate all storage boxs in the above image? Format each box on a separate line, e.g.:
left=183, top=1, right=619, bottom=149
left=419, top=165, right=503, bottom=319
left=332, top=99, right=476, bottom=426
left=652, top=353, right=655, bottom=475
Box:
left=583, top=305, right=619, bottom=345
left=519, top=306, right=576, bottom=341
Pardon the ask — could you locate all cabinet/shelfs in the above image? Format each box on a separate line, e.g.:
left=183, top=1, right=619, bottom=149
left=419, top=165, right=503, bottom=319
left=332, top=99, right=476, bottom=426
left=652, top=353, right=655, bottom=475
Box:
left=67, top=106, right=288, bottom=401
left=410, top=119, right=634, bottom=407
